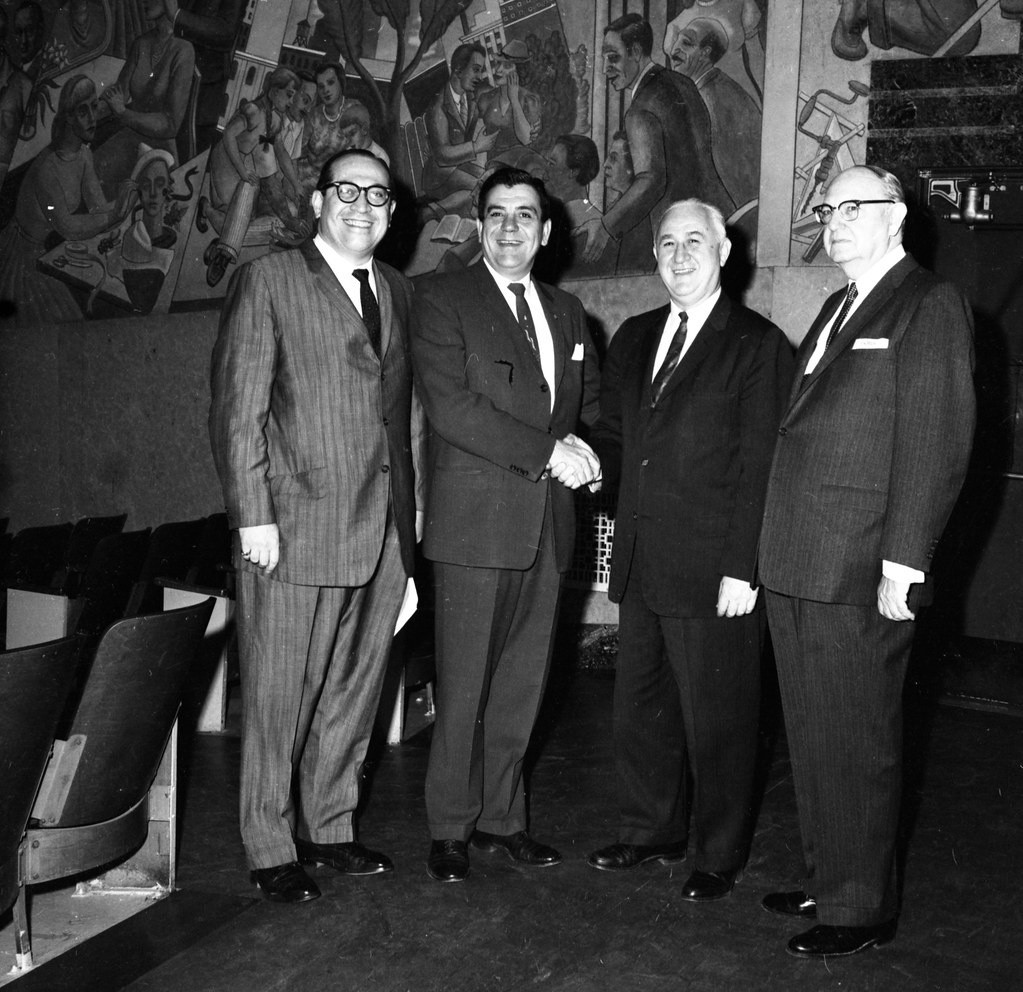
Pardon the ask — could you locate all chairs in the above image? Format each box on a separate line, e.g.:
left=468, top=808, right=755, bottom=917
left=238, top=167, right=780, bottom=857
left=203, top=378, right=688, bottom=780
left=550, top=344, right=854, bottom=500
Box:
left=0, top=474, right=1023, bottom=969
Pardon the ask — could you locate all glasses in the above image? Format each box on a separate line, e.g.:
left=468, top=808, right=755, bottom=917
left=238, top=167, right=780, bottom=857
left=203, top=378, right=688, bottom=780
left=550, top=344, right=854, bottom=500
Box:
left=322, top=182, right=394, bottom=208
left=812, top=200, right=894, bottom=222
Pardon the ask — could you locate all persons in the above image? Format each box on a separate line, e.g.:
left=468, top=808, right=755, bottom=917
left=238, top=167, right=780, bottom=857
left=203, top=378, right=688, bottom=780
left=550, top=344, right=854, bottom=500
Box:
left=411, top=170, right=602, bottom=885
left=208, top=150, right=426, bottom=901
left=544, top=200, right=796, bottom=903
left=749, top=164, right=973, bottom=959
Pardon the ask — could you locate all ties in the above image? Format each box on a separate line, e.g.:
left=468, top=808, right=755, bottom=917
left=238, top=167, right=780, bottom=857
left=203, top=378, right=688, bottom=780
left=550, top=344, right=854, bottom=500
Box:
left=649, top=312, right=688, bottom=409
left=825, top=282, right=859, bottom=348
left=352, top=269, right=383, bottom=359
left=509, top=283, right=539, bottom=364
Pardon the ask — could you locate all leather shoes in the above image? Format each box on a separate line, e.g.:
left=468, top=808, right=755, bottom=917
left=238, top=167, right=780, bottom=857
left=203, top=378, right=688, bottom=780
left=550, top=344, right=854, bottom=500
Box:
left=250, top=862, right=320, bottom=905
left=784, top=923, right=899, bottom=959
left=588, top=840, right=685, bottom=874
left=427, top=839, right=470, bottom=884
left=681, top=869, right=743, bottom=901
left=760, top=890, right=819, bottom=919
left=471, top=826, right=562, bottom=868
left=295, top=834, right=394, bottom=877
